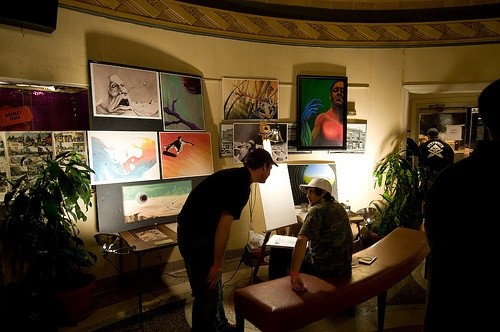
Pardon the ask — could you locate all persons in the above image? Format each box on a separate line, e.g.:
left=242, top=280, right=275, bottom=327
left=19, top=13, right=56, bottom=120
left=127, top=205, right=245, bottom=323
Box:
left=421, top=78, right=500, bottom=332
left=290, top=177, right=353, bottom=291
left=176, top=148, right=279, bottom=332
left=418, top=127, right=454, bottom=231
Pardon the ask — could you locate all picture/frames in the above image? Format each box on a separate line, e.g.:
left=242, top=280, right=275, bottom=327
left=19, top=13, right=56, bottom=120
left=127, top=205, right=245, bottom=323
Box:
left=297, top=74, right=348, bottom=149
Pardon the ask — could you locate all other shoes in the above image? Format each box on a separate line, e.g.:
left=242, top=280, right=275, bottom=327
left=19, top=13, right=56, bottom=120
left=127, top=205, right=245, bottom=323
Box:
left=224, top=324, right=238, bottom=332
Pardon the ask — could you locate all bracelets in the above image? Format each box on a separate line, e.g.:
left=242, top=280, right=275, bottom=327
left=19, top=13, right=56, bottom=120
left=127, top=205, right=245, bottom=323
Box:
left=290, top=270, right=299, bottom=275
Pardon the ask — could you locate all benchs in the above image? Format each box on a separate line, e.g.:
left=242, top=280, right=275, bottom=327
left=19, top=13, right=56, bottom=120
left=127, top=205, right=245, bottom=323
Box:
left=234, top=228, right=432, bottom=332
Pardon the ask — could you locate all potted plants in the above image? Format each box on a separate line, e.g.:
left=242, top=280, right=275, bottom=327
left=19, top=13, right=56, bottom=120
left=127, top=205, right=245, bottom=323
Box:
left=0, top=151, right=96, bottom=322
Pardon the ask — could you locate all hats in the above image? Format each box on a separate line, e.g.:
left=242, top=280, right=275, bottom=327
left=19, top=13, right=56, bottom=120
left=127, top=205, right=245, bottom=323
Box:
left=247, top=148, right=278, bottom=168
left=427, top=127, right=439, bottom=137
left=299, top=178, right=332, bottom=194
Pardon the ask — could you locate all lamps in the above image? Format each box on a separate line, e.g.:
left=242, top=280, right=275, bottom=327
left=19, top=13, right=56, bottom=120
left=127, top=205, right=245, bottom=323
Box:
left=254, top=111, right=283, bottom=142
